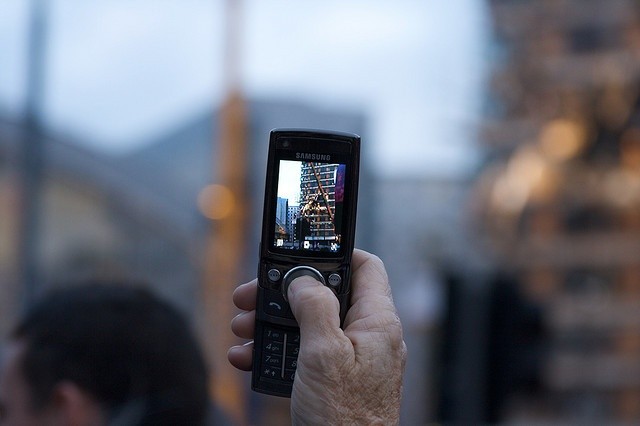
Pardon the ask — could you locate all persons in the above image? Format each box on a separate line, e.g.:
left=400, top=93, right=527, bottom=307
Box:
left=0, top=279, right=211, bottom=426
left=229, top=248, right=408, bottom=426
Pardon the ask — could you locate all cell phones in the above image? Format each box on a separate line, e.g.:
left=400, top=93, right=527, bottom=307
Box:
left=250, top=126, right=360, bottom=398
